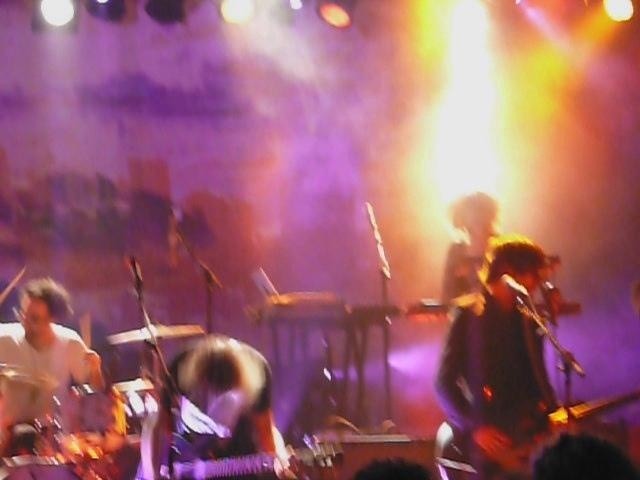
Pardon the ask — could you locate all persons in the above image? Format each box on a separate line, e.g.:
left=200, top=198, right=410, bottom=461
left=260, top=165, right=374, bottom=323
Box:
left=431, top=235, right=548, bottom=480
left=354, top=457, right=431, bottom=480
left=533, top=431, right=640, bottom=480
left=0, top=276, right=106, bottom=456
left=140, top=334, right=290, bottom=479
left=443, top=193, right=499, bottom=305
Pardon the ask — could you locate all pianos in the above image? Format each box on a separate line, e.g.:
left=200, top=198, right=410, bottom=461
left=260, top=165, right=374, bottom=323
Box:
left=252, top=299, right=399, bottom=330
left=410, top=297, right=581, bottom=316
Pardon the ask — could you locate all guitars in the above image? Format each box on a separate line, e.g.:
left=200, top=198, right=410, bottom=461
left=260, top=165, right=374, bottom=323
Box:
left=135, top=431, right=345, bottom=479
left=435, top=385, right=639, bottom=479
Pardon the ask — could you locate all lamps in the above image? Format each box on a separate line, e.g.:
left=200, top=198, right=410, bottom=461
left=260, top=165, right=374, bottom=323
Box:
left=146, top=0, right=196, bottom=24
left=85, top=0, right=138, bottom=22
left=316, top=0, right=355, bottom=28
left=604, top=0, right=634, bottom=21
left=31, top=0, right=79, bottom=32
left=218, top=0, right=253, bottom=24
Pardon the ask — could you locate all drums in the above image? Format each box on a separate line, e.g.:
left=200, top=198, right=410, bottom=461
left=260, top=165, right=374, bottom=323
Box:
left=3, top=463, right=76, bottom=480
left=112, top=377, right=154, bottom=424
left=1, top=362, right=59, bottom=458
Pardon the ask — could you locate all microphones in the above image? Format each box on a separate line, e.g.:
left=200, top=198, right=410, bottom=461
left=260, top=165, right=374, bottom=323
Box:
left=501, top=273, right=530, bottom=297
left=123, top=252, right=144, bottom=282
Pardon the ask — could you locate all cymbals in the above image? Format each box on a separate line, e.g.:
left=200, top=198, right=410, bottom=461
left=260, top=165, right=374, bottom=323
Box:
left=109, top=323, right=208, bottom=347
